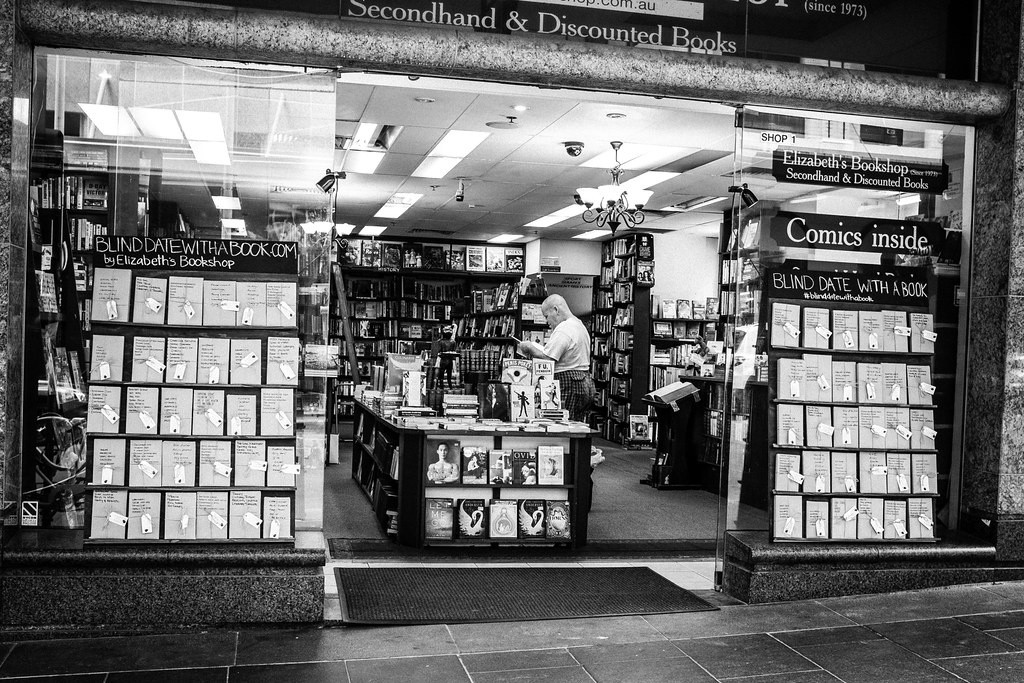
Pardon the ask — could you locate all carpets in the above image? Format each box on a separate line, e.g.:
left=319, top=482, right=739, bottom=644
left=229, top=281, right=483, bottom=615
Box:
left=332, top=567, right=722, bottom=625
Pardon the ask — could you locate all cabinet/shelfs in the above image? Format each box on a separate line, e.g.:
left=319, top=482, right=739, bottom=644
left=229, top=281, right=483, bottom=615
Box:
left=24, top=109, right=961, bottom=553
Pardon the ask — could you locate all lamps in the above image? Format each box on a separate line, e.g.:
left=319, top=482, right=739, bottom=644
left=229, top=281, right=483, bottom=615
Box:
left=574, top=140, right=655, bottom=238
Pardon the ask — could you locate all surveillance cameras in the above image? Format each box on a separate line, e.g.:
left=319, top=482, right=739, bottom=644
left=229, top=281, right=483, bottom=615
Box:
left=574, top=195, right=584, bottom=206
left=455, top=178, right=466, bottom=202
left=565, top=142, right=585, bottom=157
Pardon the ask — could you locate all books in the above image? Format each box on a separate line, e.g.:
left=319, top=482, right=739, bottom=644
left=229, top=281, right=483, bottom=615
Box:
left=28, top=170, right=113, bottom=478
left=648, top=290, right=762, bottom=472
left=426, top=439, right=571, bottom=539
left=590, top=237, right=634, bottom=446
left=348, top=414, right=400, bottom=536
left=136, top=192, right=196, bottom=240
left=721, top=222, right=760, bottom=284
left=296, top=232, right=590, bottom=434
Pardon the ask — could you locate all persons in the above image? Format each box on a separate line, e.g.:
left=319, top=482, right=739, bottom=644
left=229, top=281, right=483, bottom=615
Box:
left=426, top=443, right=459, bottom=484
left=519, top=294, right=595, bottom=422
left=638, top=271, right=654, bottom=281
